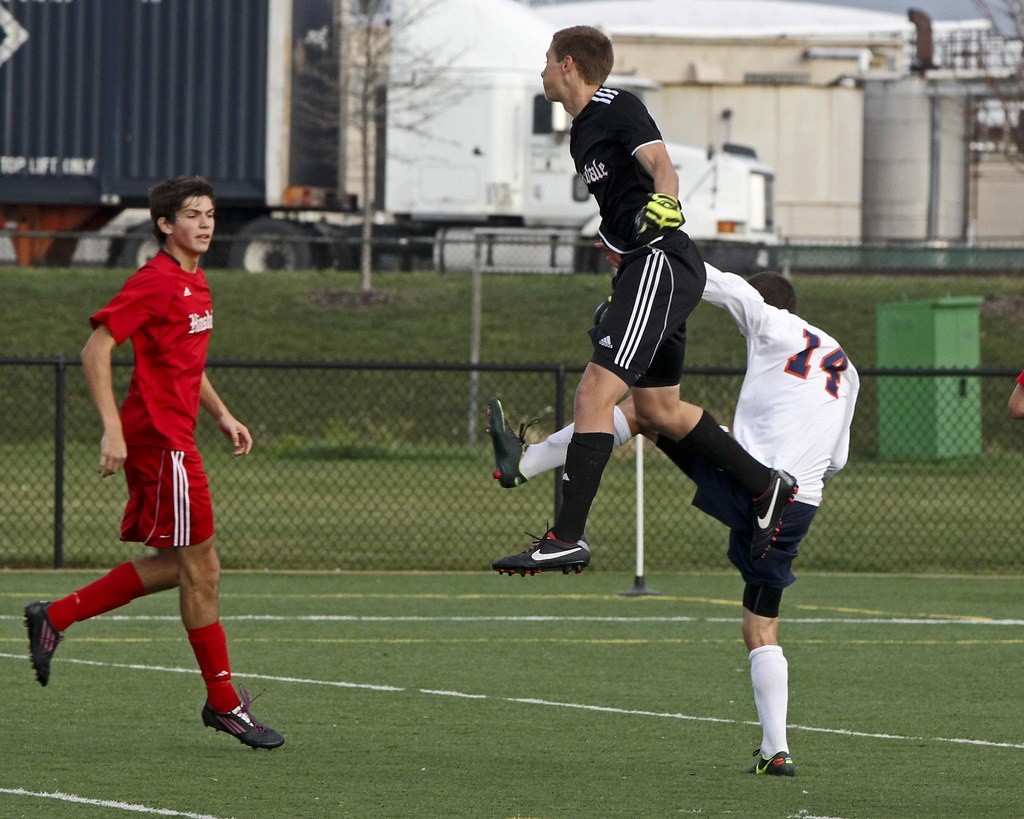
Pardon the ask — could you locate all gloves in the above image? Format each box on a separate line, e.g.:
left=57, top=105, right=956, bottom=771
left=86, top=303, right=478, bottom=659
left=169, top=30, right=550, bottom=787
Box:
left=634, top=193, right=686, bottom=241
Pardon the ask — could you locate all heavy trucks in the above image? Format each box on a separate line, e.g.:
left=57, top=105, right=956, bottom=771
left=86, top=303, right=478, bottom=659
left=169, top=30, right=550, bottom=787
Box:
left=0, top=0, right=794, bottom=273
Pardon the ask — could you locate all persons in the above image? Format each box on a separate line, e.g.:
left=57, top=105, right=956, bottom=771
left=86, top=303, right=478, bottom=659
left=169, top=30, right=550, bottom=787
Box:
left=1007, top=367, right=1024, bottom=422
left=484, top=27, right=861, bottom=776
left=24, top=178, right=286, bottom=750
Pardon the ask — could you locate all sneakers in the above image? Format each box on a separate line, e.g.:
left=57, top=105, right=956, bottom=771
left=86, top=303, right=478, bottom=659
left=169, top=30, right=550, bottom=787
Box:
left=745, top=748, right=794, bottom=777
left=201, top=682, right=284, bottom=751
left=483, top=398, right=542, bottom=488
left=748, top=468, right=799, bottom=565
left=23, top=601, right=64, bottom=688
left=491, top=519, right=591, bottom=577
